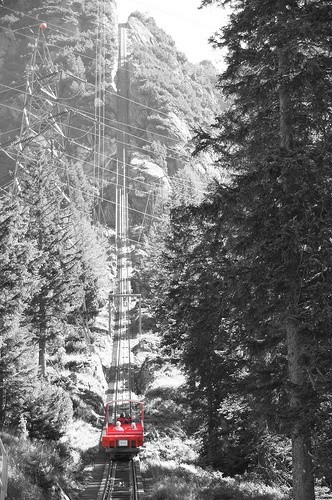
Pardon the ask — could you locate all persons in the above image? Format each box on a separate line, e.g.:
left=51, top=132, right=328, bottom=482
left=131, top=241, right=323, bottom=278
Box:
left=117, top=413, right=128, bottom=423
left=130, top=423, right=139, bottom=431
left=112, top=420, right=126, bottom=430
left=125, top=413, right=132, bottom=423
